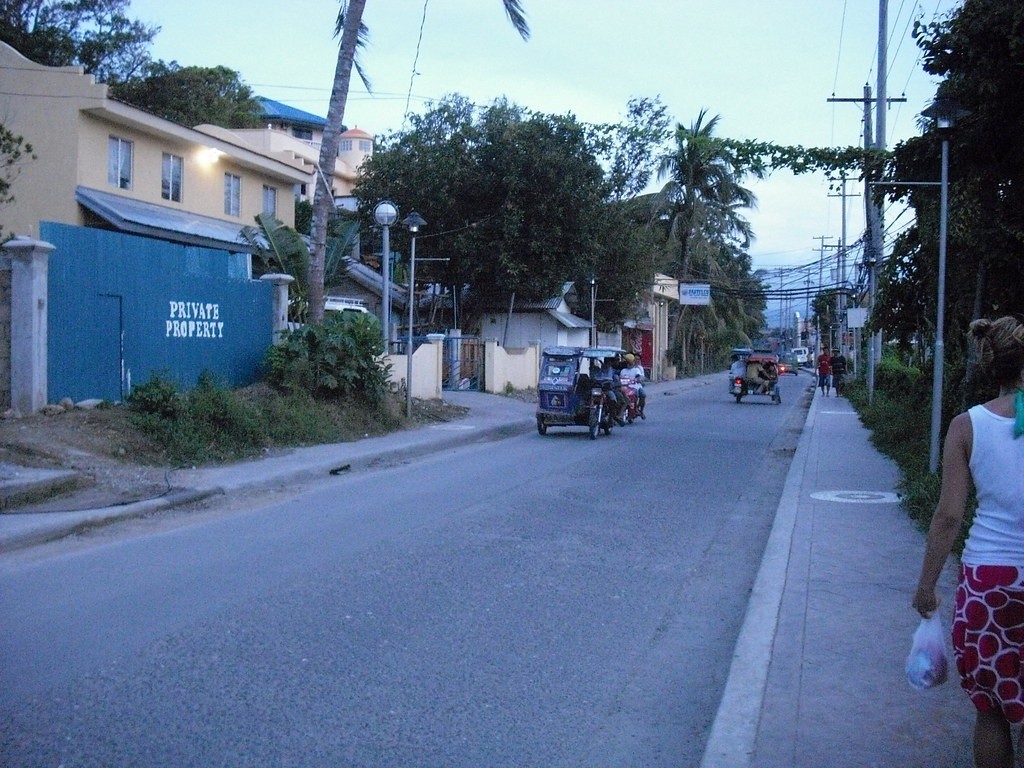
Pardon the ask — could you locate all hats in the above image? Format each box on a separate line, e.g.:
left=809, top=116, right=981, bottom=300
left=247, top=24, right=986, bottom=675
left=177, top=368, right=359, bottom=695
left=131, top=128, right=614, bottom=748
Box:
left=831, top=347, right=839, bottom=352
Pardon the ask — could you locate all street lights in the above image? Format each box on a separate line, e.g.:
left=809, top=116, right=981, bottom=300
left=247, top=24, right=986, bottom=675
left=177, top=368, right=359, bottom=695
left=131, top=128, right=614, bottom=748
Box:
left=585, top=273, right=599, bottom=350
left=372, top=200, right=401, bottom=358
left=404, top=211, right=428, bottom=419
left=922, top=97, right=977, bottom=471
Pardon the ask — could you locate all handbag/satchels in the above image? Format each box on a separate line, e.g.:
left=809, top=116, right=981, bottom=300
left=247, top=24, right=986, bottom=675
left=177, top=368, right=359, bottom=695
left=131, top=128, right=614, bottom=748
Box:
left=906, top=608, right=950, bottom=690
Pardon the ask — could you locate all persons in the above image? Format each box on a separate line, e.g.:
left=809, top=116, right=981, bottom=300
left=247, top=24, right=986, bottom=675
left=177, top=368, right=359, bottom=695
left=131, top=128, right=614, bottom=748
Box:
left=911, top=316, right=1024, bottom=768
left=729, top=355, right=777, bottom=395
left=807, top=351, right=814, bottom=368
left=590, top=352, right=647, bottom=427
left=815, top=347, right=846, bottom=397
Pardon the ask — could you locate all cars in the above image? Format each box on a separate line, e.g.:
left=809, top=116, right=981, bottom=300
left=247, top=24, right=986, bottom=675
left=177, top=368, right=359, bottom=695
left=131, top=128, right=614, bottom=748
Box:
left=776, top=346, right=811, bottom=376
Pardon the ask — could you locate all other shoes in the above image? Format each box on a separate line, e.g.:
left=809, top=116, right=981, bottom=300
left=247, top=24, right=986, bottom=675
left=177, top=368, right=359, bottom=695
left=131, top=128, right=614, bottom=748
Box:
left=826, top=394, right=829, bottom=397
left=821, top=394, right=824, bottom=397
left=641, top=411, right=646, bottom=421
left=835, top=395, right=839, bottom=398
left=613, top=416, right=624, bottom=427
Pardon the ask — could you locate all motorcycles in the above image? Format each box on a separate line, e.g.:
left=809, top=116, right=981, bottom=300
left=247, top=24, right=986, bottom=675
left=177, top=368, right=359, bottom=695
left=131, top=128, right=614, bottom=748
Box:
left=614, top=374, right=642, bottom=424
left=728, top=348, right=782, bottom=405
left=537, top=346, right=627, bottom=440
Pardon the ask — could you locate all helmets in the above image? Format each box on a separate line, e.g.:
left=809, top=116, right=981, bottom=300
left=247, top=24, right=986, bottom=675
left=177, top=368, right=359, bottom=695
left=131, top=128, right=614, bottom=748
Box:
left=623, top=353, right=635, bottom=363
